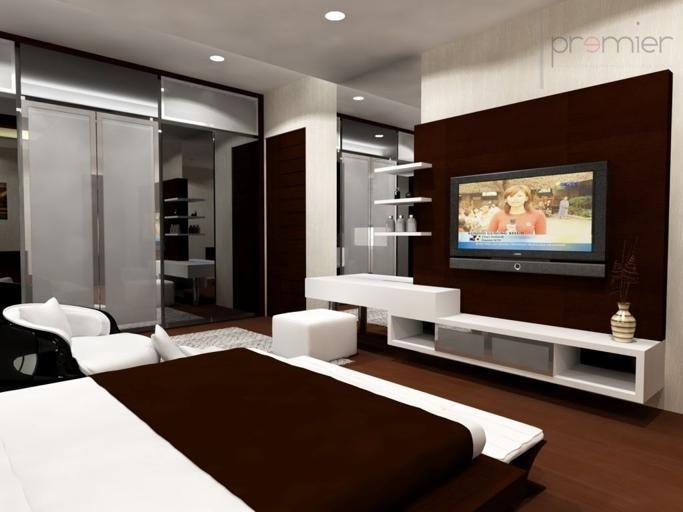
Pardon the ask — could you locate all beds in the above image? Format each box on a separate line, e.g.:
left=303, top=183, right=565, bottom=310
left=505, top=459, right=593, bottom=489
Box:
left=0, top=338, right=536, bottom=510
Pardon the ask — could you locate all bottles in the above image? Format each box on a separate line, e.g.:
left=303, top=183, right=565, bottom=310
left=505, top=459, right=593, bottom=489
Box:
left=169, top=209, right=200, bottom=234
left=385, top=213, right=416, bottom=232
left=394, top=189, right=400, bottom=199
left=406, top=192, right=412, bottom=197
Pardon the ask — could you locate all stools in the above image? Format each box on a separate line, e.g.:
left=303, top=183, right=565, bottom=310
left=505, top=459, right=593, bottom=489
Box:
left=161, top=278, right=177, bottom=309
left=267, top=307, right=362, bottom=363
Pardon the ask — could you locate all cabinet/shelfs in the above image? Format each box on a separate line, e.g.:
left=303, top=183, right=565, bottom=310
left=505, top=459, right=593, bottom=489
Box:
left=160, top=193, right=206, bottom=237
left=370, top=157, right=436, bottom=243
left=162, top=255, right=214, bottom=308
left=296, top=267, right=670, bottom=407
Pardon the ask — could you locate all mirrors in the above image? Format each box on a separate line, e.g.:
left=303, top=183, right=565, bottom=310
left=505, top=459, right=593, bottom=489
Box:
left=155, top=118, right=267, bottom=332
left=0, top=89, right=28, bottom=331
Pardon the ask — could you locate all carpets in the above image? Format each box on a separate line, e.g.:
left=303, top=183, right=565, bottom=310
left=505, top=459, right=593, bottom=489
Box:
left=151, top=326, right=356, bottom=372
left=161, top=306, right=207, bottom=330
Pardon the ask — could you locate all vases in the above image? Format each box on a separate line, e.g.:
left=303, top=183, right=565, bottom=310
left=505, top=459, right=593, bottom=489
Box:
left=606, top=300, right=639, bottom=346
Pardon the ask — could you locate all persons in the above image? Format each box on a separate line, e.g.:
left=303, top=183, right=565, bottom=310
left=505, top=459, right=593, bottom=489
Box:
left=461, top=185, right=569, bottom=235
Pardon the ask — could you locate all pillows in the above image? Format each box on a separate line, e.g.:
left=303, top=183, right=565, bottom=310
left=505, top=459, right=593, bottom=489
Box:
left=32, top=296, right=73, bottom=339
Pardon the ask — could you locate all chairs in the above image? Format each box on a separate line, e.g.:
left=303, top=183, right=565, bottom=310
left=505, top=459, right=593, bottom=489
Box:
left=1, top=295, right=163, bottom=392
left=0, top=274, right=21, bottom=313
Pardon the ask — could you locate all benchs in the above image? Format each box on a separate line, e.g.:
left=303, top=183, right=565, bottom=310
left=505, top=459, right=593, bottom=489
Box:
left=282, top=349, right=547, bottom=496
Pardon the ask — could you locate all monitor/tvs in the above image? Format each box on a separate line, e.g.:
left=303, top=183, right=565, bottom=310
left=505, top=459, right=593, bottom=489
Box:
left=449, top=159, right=609, bottom=278
